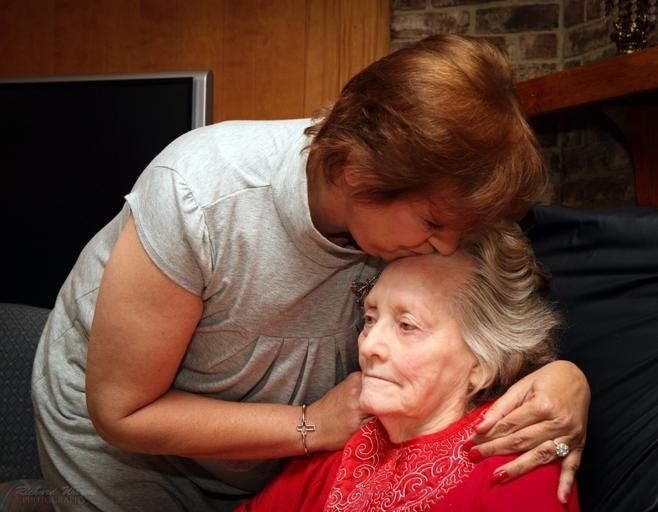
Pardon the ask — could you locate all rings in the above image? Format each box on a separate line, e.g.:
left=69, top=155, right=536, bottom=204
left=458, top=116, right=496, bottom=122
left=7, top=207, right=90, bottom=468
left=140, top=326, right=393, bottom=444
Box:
left=552, top=438, right=570, bottom=457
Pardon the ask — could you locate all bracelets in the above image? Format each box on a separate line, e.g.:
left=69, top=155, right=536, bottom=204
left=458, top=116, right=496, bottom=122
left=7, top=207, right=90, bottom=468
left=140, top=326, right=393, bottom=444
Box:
left=294, top=402, right=317, bottom=456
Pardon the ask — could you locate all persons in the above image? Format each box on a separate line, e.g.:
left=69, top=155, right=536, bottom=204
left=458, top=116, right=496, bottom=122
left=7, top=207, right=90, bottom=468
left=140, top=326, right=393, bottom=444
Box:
left=233, top=216, right=591, bottom=512
left=27, top=33, right=591, bottom=512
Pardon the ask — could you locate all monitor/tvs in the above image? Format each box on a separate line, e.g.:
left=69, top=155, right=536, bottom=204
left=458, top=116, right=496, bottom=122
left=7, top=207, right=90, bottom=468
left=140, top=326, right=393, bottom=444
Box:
left=0, top=69, right=213, bottom=307
